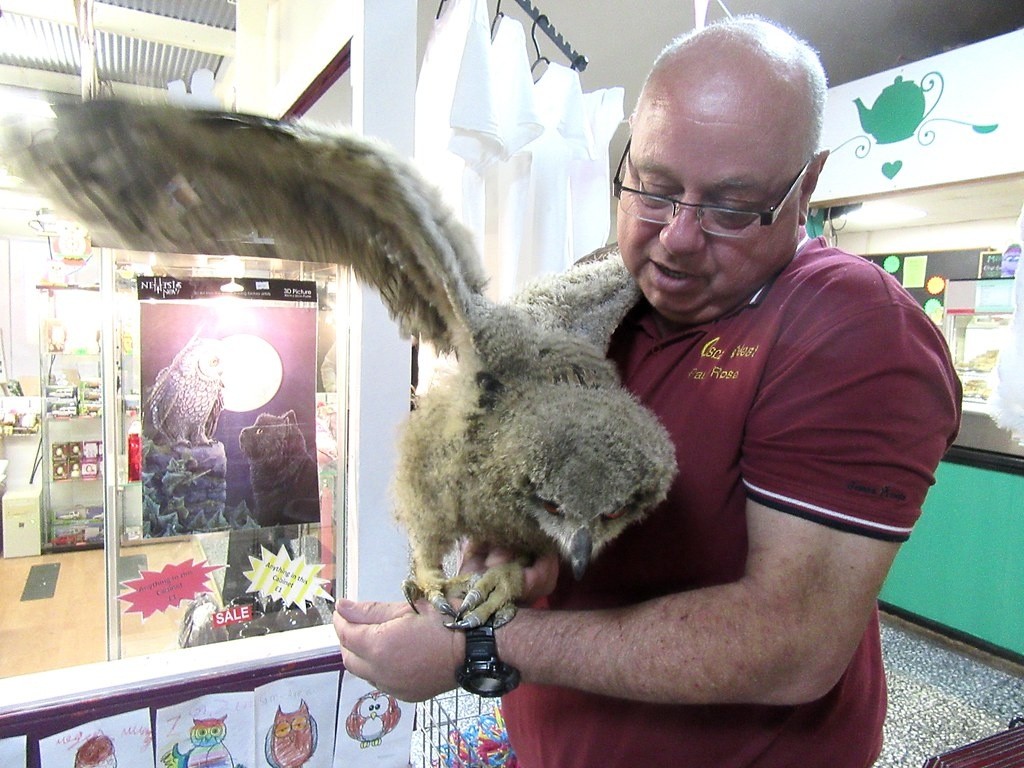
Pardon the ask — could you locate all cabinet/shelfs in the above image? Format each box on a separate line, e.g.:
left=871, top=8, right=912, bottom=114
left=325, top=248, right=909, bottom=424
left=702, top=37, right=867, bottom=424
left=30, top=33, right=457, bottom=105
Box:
left=39, top=284, right=110, bottom=548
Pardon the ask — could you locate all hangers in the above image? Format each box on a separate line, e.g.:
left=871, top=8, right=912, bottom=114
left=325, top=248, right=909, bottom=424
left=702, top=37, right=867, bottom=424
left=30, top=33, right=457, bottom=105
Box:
left=437, top=2, right=590, bottom=89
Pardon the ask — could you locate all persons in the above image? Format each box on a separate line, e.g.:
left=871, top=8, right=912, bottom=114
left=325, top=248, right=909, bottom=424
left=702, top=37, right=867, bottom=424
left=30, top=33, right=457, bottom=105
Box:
left=325, top=16, right=965, bottom=768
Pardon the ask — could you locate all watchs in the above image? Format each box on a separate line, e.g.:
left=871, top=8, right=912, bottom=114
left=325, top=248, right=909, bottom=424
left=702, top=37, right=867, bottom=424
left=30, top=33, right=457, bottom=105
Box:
left=450, top=589, right=519, bottom=699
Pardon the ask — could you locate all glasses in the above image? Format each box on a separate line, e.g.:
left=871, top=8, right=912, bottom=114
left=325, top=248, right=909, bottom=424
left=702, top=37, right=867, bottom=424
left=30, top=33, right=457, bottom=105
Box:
left=612, top=132, right=811, bottom=239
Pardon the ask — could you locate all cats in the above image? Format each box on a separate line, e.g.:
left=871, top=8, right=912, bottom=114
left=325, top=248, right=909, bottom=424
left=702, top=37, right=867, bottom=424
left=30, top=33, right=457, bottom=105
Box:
left=238, top=410, right=320, bottom=527
